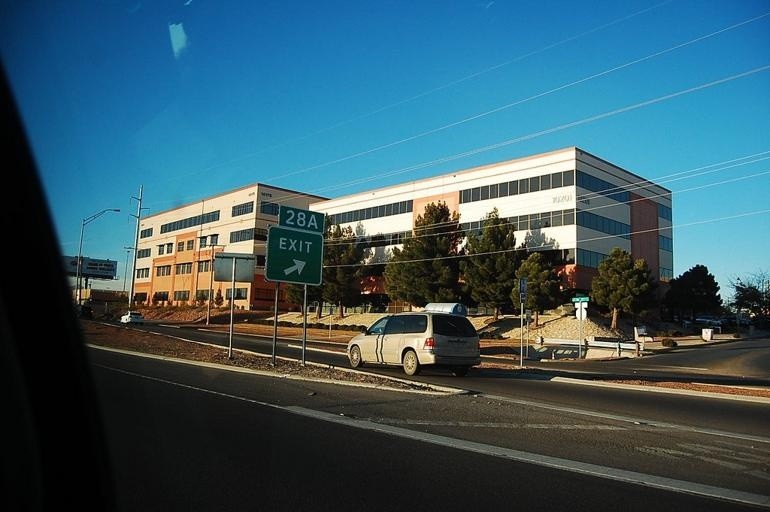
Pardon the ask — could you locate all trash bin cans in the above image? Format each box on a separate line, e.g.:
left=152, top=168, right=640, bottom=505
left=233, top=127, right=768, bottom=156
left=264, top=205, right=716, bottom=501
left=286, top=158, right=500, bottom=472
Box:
left=703, top=329, right=714, bottom=341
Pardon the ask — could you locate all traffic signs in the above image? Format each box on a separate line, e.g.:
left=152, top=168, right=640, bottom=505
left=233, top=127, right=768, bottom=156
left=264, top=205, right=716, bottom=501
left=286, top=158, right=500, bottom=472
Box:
left=264, top=223, right=326, bottom=284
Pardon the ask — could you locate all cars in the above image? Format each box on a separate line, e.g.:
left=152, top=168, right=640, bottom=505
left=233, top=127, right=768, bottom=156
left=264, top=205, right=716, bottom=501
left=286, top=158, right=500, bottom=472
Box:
left=120, top=310, right=145, bottom=325
left=347, top=309, right=482, bottom=377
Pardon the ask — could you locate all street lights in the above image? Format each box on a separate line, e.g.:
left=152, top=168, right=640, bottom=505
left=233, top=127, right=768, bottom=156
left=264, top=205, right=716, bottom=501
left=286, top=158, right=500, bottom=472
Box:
left=74, top=209, right=120, bottom=306
